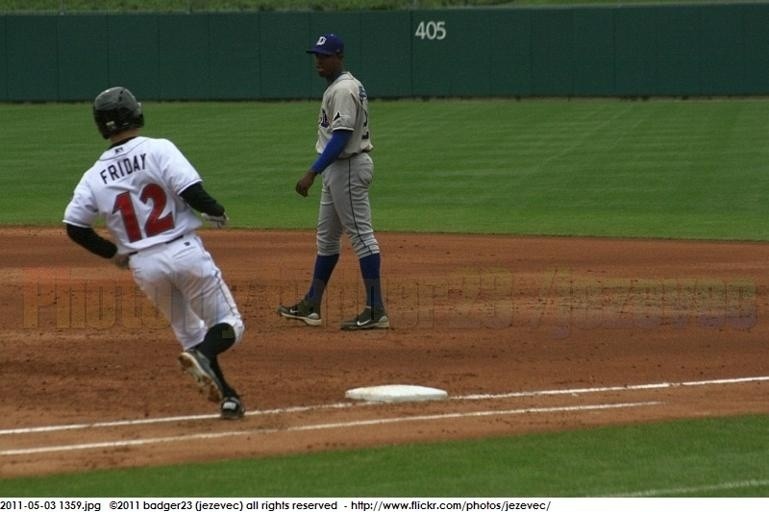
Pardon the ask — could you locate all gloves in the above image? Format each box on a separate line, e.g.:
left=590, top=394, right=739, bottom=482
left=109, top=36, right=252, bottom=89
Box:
left=201, top=211, right=228, bottom=229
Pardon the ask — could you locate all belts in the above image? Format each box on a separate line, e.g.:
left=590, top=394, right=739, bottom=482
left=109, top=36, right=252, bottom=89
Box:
left=129, top=236, right=183, bottom=255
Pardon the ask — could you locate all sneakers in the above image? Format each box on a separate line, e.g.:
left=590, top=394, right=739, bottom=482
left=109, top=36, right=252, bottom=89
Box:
left=341, top=304, right=390, bottom=330
left=178, top=347, right=224, bottom=402
left=279, top=301, right=322, bottom=326
left=220, top=395, right=246, bottom=418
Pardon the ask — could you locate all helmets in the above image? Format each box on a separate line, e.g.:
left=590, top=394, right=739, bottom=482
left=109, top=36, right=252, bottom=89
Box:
left=92, top=87, right=144, bottom=139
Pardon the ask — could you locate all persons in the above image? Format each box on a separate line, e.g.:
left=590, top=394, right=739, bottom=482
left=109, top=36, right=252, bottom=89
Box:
left=278, top=33, right=391, bottom=331
left=62, top=86, right=246, bottom=420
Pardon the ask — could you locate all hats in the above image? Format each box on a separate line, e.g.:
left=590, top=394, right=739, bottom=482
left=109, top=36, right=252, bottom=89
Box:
left=306, top=33, right=344, bottom=56
